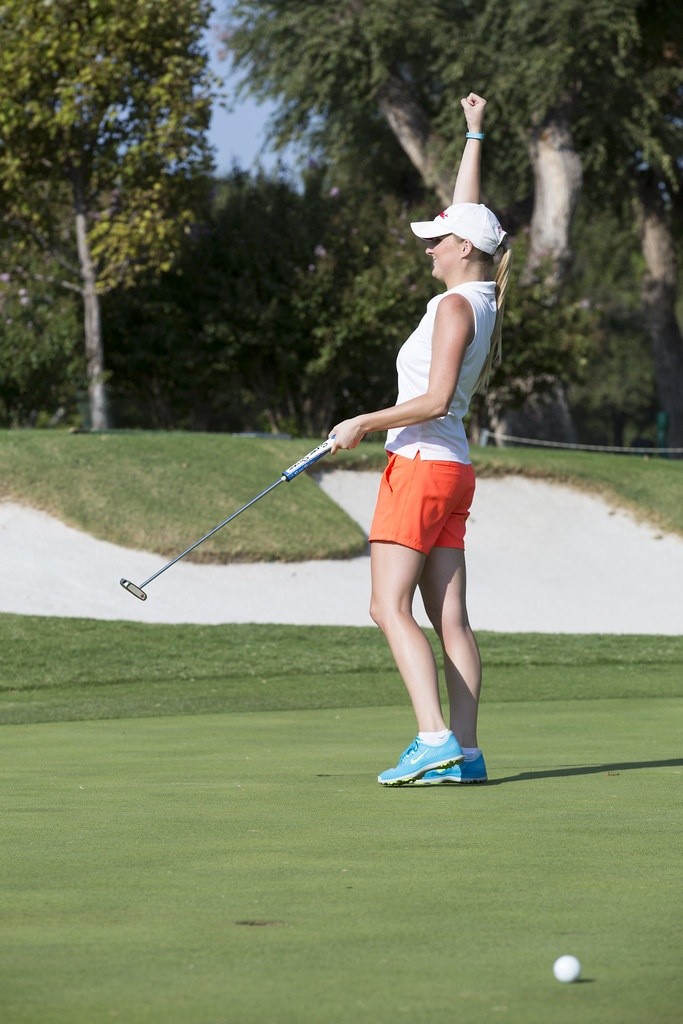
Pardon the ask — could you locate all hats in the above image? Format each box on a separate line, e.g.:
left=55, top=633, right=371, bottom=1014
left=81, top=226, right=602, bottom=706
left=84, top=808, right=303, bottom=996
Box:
left=409, top=201, right=507, bottom=256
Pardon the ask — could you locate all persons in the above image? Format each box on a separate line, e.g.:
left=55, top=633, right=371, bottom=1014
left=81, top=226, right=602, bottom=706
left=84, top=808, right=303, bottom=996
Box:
left=329, top=92, right=513, bottom=786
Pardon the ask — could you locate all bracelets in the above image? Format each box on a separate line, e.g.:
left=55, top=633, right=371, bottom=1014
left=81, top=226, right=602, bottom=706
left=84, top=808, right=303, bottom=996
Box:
left=465, top=132, right=485, bottom=140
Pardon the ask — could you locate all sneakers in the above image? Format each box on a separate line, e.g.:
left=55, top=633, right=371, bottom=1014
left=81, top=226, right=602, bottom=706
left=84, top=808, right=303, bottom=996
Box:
left=412, top=748, right=489, bottom=784
left=377, top=729, right=465, bottom=786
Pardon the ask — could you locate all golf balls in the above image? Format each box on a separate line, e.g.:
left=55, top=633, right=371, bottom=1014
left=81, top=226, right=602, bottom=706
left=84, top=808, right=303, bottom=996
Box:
left=552, top=954, right=582, bottom=982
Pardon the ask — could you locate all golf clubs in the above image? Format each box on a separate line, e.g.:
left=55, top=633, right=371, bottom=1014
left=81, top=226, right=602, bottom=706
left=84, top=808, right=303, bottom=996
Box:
left=115, top=436, right=333, bottom=601
